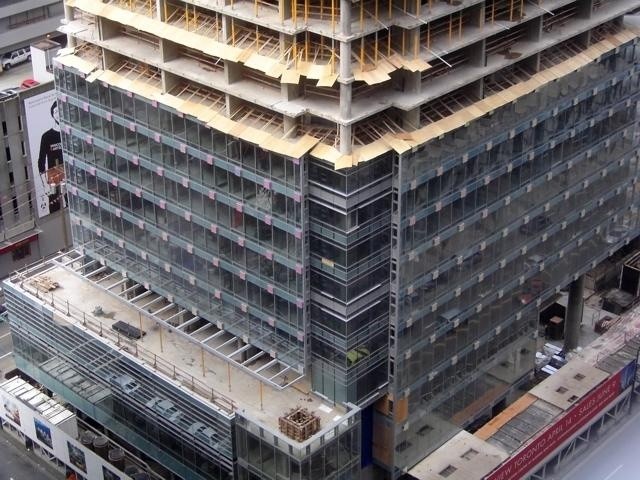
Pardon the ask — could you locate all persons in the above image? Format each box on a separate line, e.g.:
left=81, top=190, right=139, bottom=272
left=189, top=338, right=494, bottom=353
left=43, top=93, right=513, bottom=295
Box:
left=38, top=101, right=65, bottom=214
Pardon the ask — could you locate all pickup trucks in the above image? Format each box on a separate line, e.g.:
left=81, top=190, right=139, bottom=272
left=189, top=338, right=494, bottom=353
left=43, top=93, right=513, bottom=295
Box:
left=1, top=48, right=31, bottom=70
